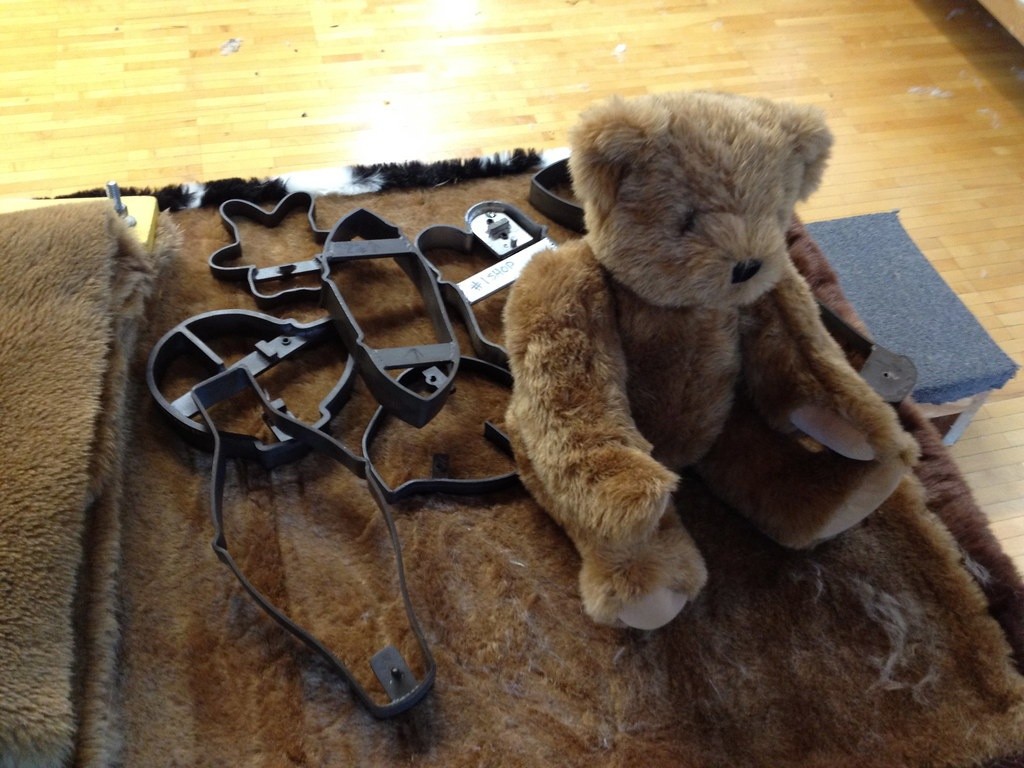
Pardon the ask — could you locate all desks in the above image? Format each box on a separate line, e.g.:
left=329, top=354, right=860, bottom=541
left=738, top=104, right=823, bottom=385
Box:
left=0, top=146, right=1024, bottom=768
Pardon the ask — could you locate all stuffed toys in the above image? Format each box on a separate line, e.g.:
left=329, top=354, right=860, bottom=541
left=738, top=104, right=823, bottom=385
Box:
left=502, top=89, right=917, bottom=634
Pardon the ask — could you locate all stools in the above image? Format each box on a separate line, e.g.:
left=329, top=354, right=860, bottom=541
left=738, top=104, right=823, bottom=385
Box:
left=801, top=208, right=1020, bottom=448
